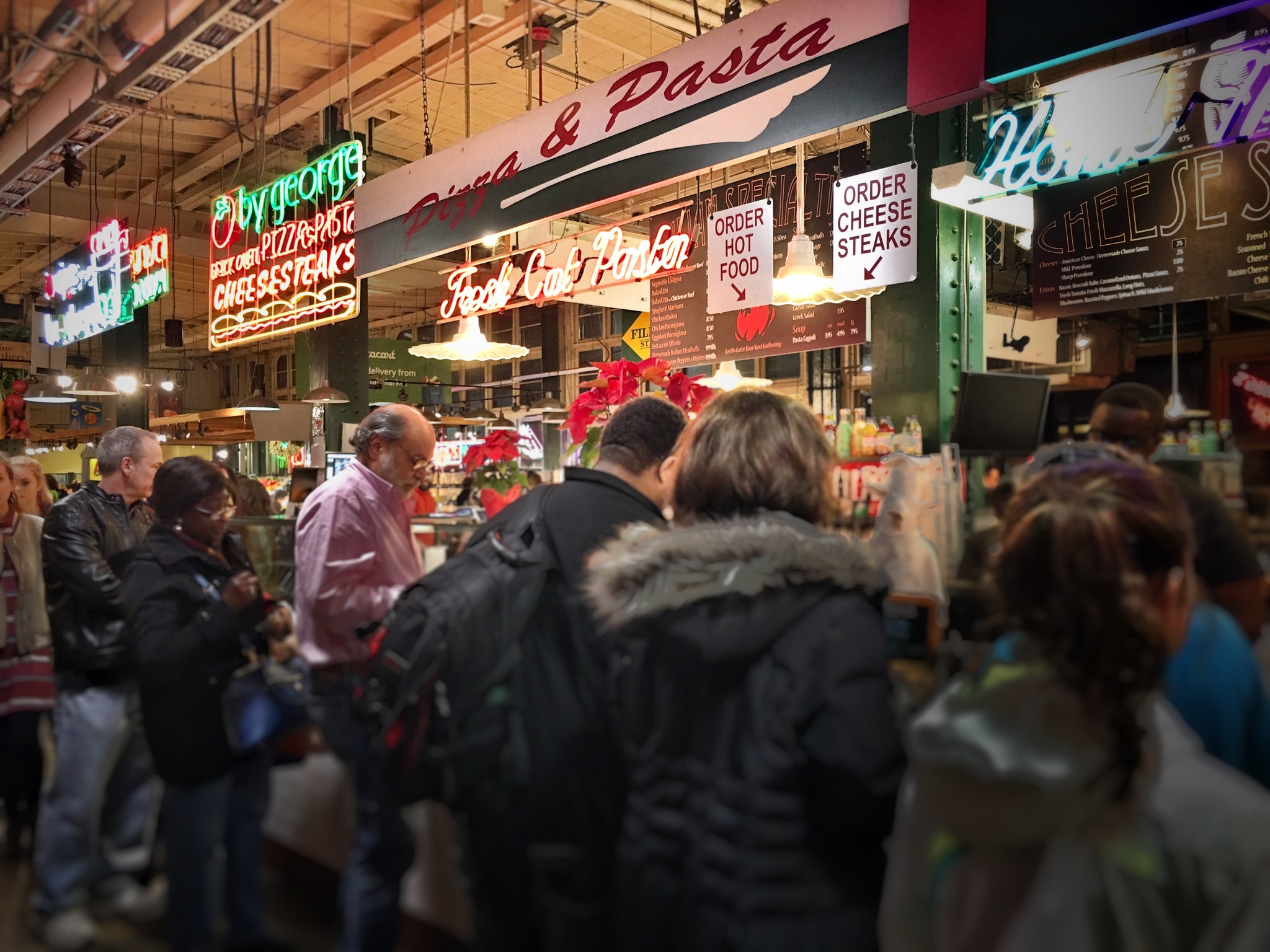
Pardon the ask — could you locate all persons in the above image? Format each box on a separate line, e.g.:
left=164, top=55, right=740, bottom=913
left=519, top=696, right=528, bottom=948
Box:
left=1012, top=438, right=1269, bottom=792
left=5, top=452, right=551, bottom=601
left=0, top=451, right=57, bottom=869
left=19, top=425, right=166, bottom=952
left=117, top=453, right=296, bottom=952
left=869, top=460, right=1269, bottom=952
left=459, top=394, right=690, bottom=952
left=291, top=400, right=439, bottom=952
left=577, top=390, right=906, bottom=951
left=1085, top=378, right=1270, bottom=649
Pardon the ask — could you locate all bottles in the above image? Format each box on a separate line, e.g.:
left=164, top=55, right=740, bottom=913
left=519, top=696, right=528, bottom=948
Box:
left=1189, top=418, right=1236, bottom=454
left=824, top=406, right=922, bottom=458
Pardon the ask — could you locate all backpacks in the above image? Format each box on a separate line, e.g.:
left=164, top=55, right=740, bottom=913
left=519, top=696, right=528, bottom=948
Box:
left=349, top=482, right=556, bottom=807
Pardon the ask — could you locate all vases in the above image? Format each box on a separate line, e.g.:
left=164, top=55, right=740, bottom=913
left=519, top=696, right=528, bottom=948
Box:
left=479, top=481, right=523, bottom=520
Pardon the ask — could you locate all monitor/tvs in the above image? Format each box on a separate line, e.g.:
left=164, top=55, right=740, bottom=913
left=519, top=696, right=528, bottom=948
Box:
left=951, top=369, right=1052, bottom=457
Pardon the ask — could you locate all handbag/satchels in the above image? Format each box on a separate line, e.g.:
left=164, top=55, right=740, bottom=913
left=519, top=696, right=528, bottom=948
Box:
left=205, top=650, right=315, bottom=755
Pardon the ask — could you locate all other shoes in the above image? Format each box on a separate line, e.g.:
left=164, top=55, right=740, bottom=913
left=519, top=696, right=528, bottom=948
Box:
left=98, top=876, right=169, bottom=923
left=228, top=935, right=299, bottom=952
left=26, top=903, right=94, bottom=950
left=1, top=820, right=34, bottom=861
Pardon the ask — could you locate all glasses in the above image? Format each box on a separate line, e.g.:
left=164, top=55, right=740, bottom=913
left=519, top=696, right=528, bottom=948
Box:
left=396, top=443, right=435, bottom=472
left=1086, top=428, right=1154, bottom=450
left=190, top=504, right=238, bottom=521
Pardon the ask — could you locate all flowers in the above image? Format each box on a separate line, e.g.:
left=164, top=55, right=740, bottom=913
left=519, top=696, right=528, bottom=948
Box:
left=463, top=430, right=531, bottom=497
left=551, top=357, right=713, bottom=466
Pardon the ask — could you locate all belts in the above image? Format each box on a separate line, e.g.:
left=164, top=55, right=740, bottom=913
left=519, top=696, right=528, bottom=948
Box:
left=309, top=662, right=373, bottom=682
